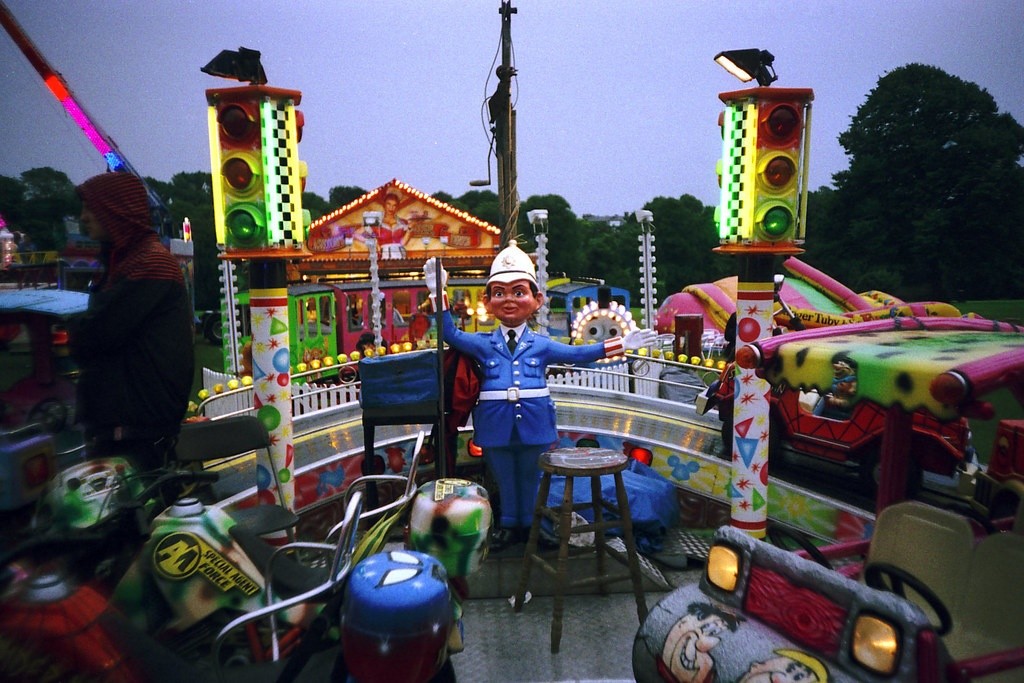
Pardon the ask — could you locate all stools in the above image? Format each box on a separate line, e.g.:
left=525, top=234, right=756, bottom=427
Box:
left=513, top=447, right=648, bottom=653
left=362, top=402, right=441, bottom=508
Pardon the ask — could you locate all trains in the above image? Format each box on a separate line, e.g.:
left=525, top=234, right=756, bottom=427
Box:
left=237, top=276, right=633, bottom=388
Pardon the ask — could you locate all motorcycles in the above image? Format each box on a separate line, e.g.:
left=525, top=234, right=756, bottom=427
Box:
left=0, top=431, right=493, bottom=683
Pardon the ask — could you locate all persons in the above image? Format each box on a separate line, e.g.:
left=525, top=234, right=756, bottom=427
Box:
left=62, top=172, right=197, bottom=640
left=423, top=239, right=659, bottom=553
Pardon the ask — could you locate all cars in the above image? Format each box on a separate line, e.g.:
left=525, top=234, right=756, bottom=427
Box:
left=632, top=420, right=1024, bottom=683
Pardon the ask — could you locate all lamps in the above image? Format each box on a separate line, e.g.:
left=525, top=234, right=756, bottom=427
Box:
left=714, top=48, right=777, bottom=88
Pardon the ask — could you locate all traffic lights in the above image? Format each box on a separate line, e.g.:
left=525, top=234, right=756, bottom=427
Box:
left=206, top=81, right=309, bottom=260
left=713, top=88, right=813, bottom=253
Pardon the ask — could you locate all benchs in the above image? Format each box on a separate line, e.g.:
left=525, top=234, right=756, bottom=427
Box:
left=860, top=501, right=1024, bottom=683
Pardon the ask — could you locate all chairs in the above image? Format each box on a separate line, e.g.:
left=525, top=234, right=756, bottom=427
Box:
left=174, top=415, right=301, bottom=565
